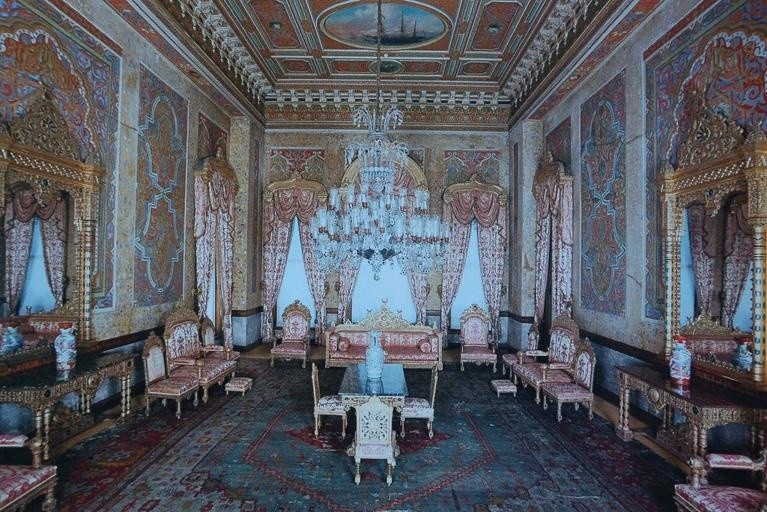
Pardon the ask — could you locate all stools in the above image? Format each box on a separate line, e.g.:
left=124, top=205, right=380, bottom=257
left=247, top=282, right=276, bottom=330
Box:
left=224, top=376, right=252, bottom=397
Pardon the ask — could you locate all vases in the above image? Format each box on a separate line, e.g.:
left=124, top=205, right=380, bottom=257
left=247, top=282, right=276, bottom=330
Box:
left=365, top=331, right=388, bottom=381
left=365, top=377, right=384, bottom=397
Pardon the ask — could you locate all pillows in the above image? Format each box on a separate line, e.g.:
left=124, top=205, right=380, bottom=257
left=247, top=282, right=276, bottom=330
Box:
left=417, top=338, right=431, bottom=353
left=338, top=336, right=350, bottom=351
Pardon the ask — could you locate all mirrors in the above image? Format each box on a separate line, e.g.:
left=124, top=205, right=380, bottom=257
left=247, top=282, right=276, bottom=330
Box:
left=0, top=88, right=106, bottom=378
left=661, top=96, right=764, bottom=394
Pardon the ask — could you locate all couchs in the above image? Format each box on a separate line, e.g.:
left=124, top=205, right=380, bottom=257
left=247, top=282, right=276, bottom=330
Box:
left=679, top=308, right=752, bottom=352
left=325, top=306, right=444, bottom=371
left=0, top=315, right=81, bottom=347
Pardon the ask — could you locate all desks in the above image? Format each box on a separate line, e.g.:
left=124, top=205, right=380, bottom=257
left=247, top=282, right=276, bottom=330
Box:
left=614, top=363, right=767, bottom=486
left=337, top=363, right=409, bottom=411
left=1, top=352, right=141, bottom=460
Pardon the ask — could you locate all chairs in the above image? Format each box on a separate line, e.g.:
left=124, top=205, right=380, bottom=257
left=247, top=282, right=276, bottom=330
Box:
left=270, top=299, right=313, bottom=368
left=1, top=433, right=58, bottom=512
left=141, top=296, right=241, bottom=420
left=458, top=304, right=598, bottom=422
left=310, top=362, right=439, bottom=488
left=674, top=451, right=767, bottom=511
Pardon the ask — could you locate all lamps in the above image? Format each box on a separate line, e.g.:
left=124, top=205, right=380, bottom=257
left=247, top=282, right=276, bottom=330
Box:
left=308, top=0, right=450, bottom=280
